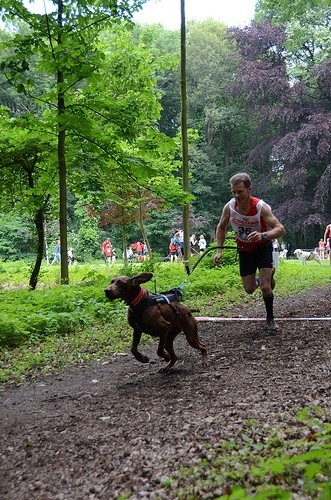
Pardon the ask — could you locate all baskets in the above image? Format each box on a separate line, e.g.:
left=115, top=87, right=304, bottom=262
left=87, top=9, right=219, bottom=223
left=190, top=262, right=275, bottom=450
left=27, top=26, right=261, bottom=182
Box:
left=255, top=270, right=275, bottom=290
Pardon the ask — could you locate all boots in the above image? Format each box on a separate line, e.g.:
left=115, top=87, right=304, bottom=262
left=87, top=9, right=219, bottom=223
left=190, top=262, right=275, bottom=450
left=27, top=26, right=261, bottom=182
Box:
left=264, top=294, right=280, bottom=333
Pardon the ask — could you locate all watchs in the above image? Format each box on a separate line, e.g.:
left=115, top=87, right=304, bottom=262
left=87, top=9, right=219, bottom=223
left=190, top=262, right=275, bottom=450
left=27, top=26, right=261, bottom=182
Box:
left=261, top=232, right=267, bottom=241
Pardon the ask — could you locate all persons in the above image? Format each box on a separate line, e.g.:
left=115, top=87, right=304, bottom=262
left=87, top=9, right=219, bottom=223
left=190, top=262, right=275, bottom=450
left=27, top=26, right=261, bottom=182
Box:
left=67, top=247, right=77, bottom=266
left=122, top=241, right=150, bottom=263
left=213, top=172, right=285, bottom=331
left=102, top=238, right=116, bottom=267
left=319, top=223, right=331, bottom=260
left=169, top=229, right=207, bottom=262
left=272, top=239, right=291, bottom=260
left=54, top=239, right=61, bottom=263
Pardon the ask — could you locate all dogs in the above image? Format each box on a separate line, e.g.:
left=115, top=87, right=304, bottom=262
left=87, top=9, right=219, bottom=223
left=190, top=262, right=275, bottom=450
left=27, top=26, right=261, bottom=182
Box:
left=104, top=273, right=208, bottom=373
left=294, top=249, right=321, bottom=266
